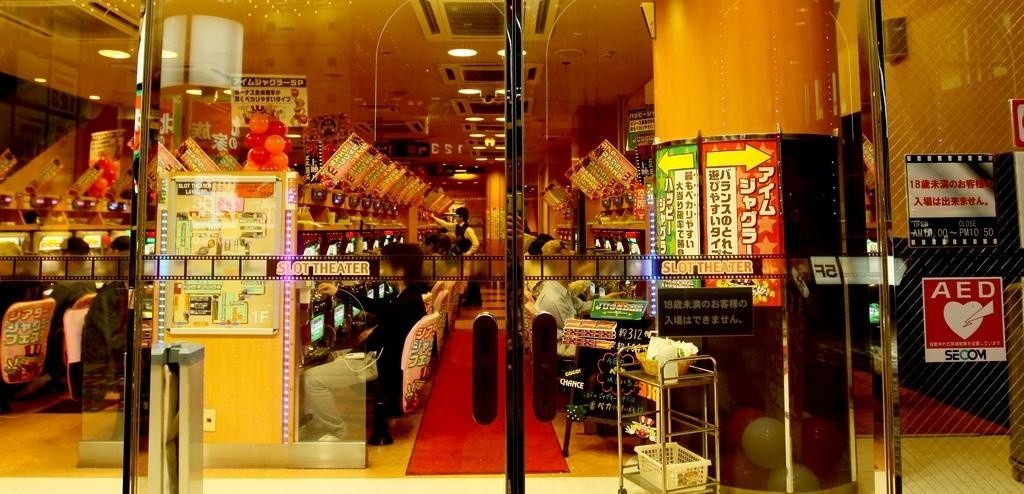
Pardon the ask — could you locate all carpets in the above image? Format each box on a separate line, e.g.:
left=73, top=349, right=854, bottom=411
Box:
left=405, top=328, right=571, bottom=475
left=0, top=364, right=125, bottom=414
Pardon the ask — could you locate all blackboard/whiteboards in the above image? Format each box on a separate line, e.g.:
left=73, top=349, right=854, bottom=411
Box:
left=571, top=313, right=651, bottom=421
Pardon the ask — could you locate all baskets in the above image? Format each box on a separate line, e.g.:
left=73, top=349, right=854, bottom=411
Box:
left=633, top=440, right=712, bottom=492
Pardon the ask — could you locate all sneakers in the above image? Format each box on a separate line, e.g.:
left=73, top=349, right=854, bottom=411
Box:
left=314, top=426, right=350, bottom=442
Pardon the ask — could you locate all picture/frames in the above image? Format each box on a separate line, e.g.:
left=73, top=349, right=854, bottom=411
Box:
left=163, top=175, right=283, bottom=336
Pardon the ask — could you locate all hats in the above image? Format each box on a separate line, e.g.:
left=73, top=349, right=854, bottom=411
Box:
left=542, top=240, right=577, bottom=257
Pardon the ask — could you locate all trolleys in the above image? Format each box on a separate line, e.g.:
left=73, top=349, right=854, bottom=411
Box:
left=608, top=342, right=721, bottom=494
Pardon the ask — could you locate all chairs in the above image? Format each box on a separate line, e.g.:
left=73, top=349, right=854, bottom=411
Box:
left=524, top=300, right=583, bottom=399
left=431, top=280, right=445, bottom=299
left=432, top=290, right=449, bottom=359
left=1, top=298, right=56, bottom=385
left=61, top=293, right=97, bottom=384
left=422, top=293, right=436, bottom=315
left=447, top=279, right=461, bottom=329
left=350, top=311, right=440, bottom=446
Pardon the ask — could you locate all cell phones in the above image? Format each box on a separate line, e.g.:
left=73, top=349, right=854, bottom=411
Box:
left=346, top=352, right=365, bottom=360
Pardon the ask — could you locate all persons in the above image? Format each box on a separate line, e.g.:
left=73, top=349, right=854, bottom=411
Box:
left=421, top=207, right=483, bottom=310
left=290, top=98, right=306, bottom=126
left=46, top=237, right=97, bottom=301
left=105, top=236, right=131, bottom=287
left=303, top=243, right=439, bottom=442
left=522, top=234, right=629, bottom=359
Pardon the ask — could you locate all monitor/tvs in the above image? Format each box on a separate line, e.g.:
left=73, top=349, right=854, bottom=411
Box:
left=352, top=306, right=361, bottom=317
left=310, top=312, right=325, bottom=342
left=378, top=283, right=385, bottom=299
left=588, top=283, right=595, bottom=296
left=367, top=289, right=375, bottom=299
left=388, top=285, right=394, bottom=292
left=599, top=287, right=605, bottom=297
left=333, top=303, right=345, bottom=328
